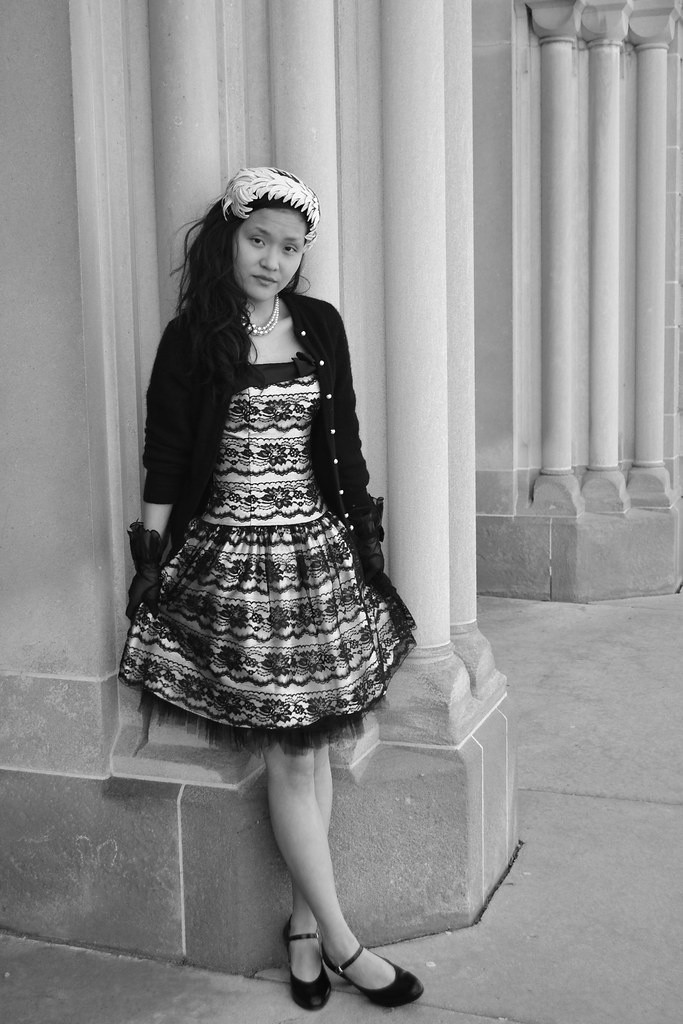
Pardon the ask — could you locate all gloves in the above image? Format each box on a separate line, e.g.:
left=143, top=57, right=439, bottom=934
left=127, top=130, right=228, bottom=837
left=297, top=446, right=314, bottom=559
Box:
left=352, top=496, right=385, bottom=578
left=126, top=521, right=166, bottom=621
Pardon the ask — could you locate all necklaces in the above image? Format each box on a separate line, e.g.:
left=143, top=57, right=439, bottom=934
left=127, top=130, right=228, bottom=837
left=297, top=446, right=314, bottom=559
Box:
left=242, top=294, right=279, bottom=336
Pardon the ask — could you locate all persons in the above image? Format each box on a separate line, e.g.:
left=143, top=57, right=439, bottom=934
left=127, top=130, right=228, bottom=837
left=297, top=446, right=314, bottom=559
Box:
left=119, top=168, right=423, bottom=1011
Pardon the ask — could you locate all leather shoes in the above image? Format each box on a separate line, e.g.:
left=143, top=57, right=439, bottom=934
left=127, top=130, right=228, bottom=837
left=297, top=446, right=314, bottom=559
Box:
left=283, top=914, right=331, bottom=1011
left=321, top=940, right=424, bottom=1007
